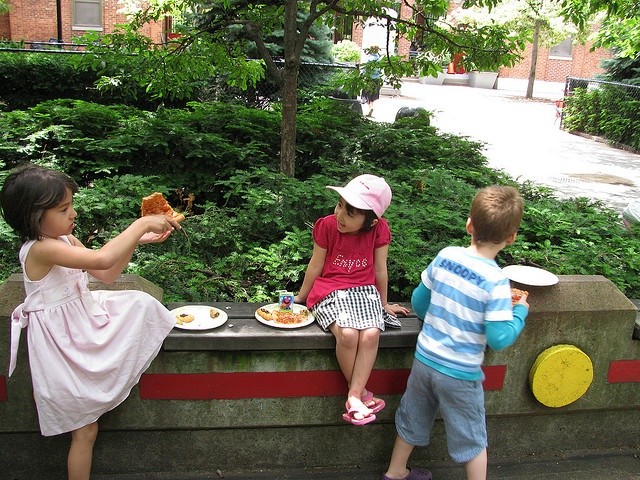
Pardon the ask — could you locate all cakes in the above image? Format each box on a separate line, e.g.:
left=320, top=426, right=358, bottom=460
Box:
left=174, top=313, right=193, bottom=323
left=256, top=308, right=271, bottom=320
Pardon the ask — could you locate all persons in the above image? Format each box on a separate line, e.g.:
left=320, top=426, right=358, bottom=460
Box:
left=0, top=166, right=181, bottom=480
left=294, top=174, right=410, bottom=426
left=383, top=185, right=529, bottom=480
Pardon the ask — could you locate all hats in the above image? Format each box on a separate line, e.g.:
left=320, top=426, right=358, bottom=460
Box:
left=324, top=174, right=393, bottom=218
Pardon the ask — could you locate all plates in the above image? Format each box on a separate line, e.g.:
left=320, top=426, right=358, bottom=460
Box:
left=255, top=303, right=316, bottom=329
left=169, top=305, right=229, bottom=331
left=501, top=265, right=559, bottom=288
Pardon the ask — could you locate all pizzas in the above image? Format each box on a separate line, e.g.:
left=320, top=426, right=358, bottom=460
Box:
left=510, top=287, right=529, bottom=305
left=140, top=191, right=185, bottom=223
left=271, top=311, right=309, bottom=324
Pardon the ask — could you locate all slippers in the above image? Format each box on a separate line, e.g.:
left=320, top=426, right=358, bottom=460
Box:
left=378, top=466, right=432, bottom=480
left=361, top=392, right=386, bottom=413
left=344, top=400, right=376, bottom=427
